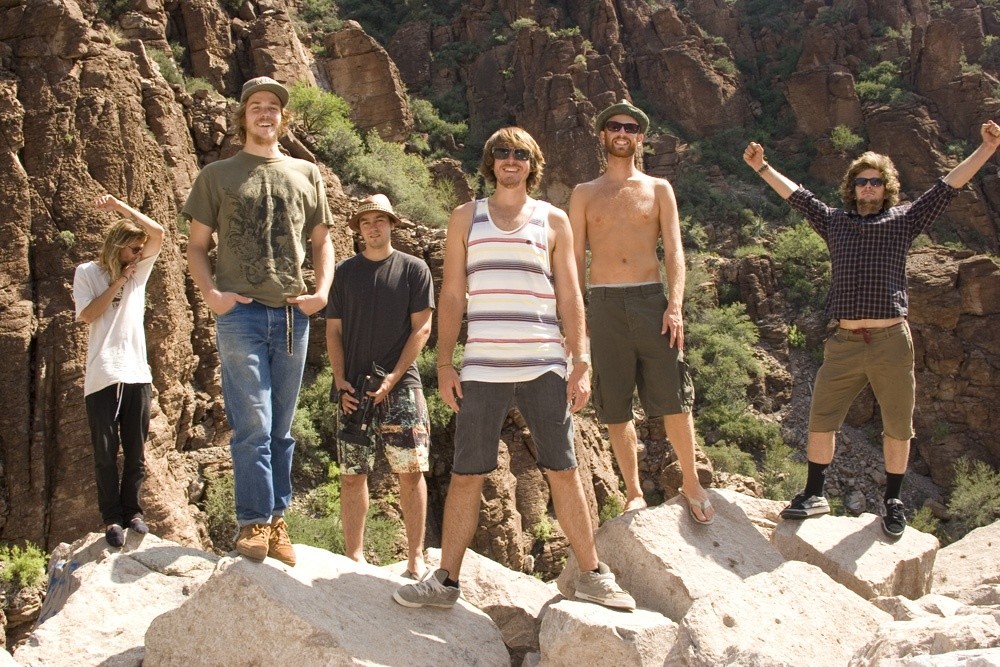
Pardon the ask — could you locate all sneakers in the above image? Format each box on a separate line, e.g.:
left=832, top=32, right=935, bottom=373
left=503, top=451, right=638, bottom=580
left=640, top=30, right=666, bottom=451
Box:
left=393, top=567, right=462, bottom=612
left=266, top=515, right=297, bottom=568
left=574, top=561, right=636, bottom=611
left=879, top=496, right=907, bottom=540
left=779, top=491, right=830, bottom=520
left=235, top=523, right=276, bottom=561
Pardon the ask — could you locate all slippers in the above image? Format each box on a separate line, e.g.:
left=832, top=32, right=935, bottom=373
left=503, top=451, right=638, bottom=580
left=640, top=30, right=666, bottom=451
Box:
left=676, top=486, right=715, bottom=525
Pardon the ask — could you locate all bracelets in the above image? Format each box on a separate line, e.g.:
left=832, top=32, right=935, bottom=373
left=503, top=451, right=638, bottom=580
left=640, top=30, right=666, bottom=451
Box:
left=120, top=272, right=128, bottom=283
left=436, top=362, right=453, bottom=370
left=756, top=165, right=769, bottom=175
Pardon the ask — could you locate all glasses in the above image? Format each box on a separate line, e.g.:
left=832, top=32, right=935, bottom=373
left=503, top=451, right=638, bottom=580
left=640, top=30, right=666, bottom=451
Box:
left=604, top=120, right=642, bottom=133
left=490, top=146, right=532, bottom=161
left=128, top=244, right=143, bottom=256
left=851, top=177, right=884, bottom=188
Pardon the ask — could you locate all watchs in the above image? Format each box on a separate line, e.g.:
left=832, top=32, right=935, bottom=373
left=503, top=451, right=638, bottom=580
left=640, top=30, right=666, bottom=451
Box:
left=572, top=353, right=592, bottom=368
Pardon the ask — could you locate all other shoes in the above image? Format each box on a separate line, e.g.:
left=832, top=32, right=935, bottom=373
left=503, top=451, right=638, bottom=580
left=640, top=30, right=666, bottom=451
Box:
left=105, top=524, right=124, bottom=548
left=128, top=518, right=150, bottom=533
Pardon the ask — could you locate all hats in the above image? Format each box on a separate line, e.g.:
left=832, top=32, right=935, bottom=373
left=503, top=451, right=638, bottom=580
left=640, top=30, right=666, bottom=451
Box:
left=347, top=192, right=401, bottom=233
left=594, top=98, right=650, bottom=135
left=239, top=75, right=290, bottom=109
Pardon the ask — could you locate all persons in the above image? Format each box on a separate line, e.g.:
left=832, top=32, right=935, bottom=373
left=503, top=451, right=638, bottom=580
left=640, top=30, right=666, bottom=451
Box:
left=325, top=195, right=437, bottom=581
left=570, top=98, right=715, bottom=526
left=738, top=120, right=1000, bottom=548
left=182, top=76, right=335, bottom=567
left=393, top=125, right=637, bottom=608
left=73, top=192, right=165, bottom=546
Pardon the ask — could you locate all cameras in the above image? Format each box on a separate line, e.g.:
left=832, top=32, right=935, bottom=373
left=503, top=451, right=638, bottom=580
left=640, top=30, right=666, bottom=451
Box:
left=339, top=373, right=382, bottom=447
